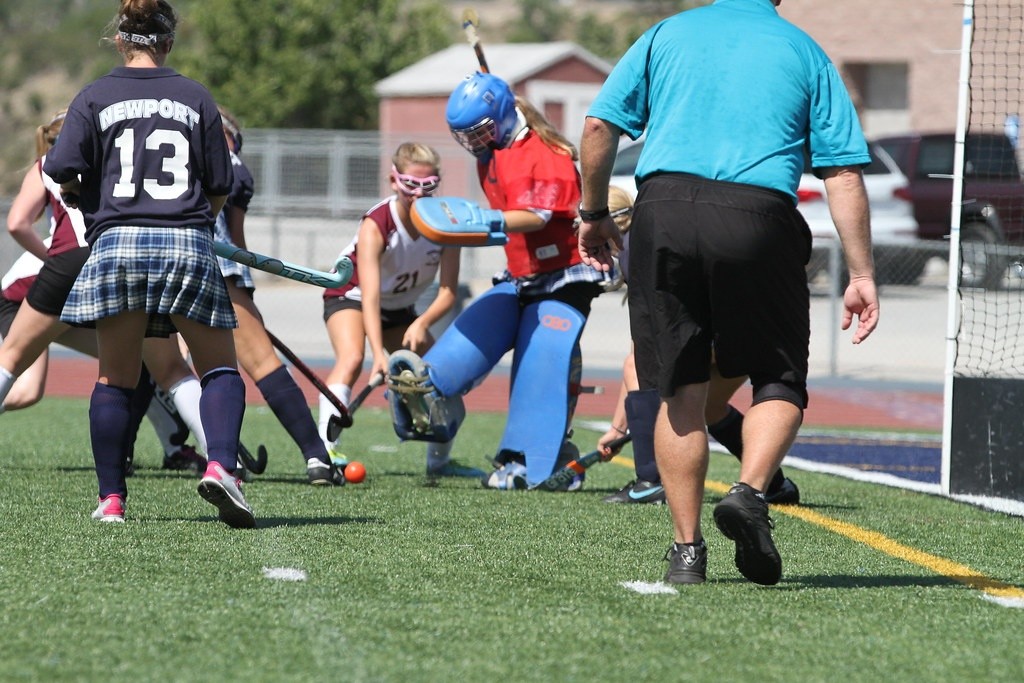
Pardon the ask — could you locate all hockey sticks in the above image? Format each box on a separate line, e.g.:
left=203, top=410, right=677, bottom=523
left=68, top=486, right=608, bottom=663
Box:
left=237, top=442, right=268, bottom=474
left=527, top=433, right=633, bottom=490
left=462, top=17, right=606, bottom=394
left=214, top=239, right=354, bottom=288
left=264, top=327, right=353, bottom=429
left=326, top=342, right=412, bottom=442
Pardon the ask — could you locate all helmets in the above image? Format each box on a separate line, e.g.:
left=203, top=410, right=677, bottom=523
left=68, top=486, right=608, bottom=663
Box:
left=446, top=73, right=516, bottom=162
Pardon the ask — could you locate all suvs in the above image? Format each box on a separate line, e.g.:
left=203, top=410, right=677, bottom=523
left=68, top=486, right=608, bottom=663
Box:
left=870, top=132, right=1024, bottom=285
left=609, top=130, right=919, bottom=284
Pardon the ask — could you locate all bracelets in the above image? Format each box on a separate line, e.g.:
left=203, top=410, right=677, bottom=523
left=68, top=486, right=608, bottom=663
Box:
left=579, top=202, right=609, bottom=221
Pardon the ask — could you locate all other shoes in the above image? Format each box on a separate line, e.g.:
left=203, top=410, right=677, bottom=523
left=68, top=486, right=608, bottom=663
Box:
left=397, top=369, right=432, bottom=435
left=482, top=460, right=527, bottom=490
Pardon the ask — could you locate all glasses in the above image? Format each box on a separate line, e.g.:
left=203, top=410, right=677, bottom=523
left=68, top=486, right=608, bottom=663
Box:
left=396, top=174, right=442, bottom=194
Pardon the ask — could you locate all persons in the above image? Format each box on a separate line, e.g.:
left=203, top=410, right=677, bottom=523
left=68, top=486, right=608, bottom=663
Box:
left=572, top=185, right=799, bottom=505
left=386, top=70, right=603, bottom=490
left=318, top=142, right=484, bottom=477
left=0, top=111, right=247, bottom=477
left=140, top=106, right=346, bottom=487
left=42, top=0, right=258, bottom=530
left=577, top=0, right=879, bottom=585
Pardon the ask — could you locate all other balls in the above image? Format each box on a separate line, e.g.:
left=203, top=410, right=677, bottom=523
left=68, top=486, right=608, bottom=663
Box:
left=345, top=462, right=366, bottom=483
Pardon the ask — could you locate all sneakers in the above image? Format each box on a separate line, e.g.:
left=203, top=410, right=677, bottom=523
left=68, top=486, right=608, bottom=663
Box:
left=713, top=480, right=782, bottom=585
left=161, top=444, right=209, bottom=473
left=762, top=476, right=801, bottom=505
left=123, top=446, right=136, bottom=475
left=307, top=457, right=347, bottom=487
left=328, top=448, right=349, bottom=466
left=425, top=459, right=488, bottom=478
left=197, top=460, right=256, bottom=529
left=601, top=478, right=667, bottom=505
left=661, top=539, right=708, bottom=583
left=91, top=494, right=125, bottom=523
